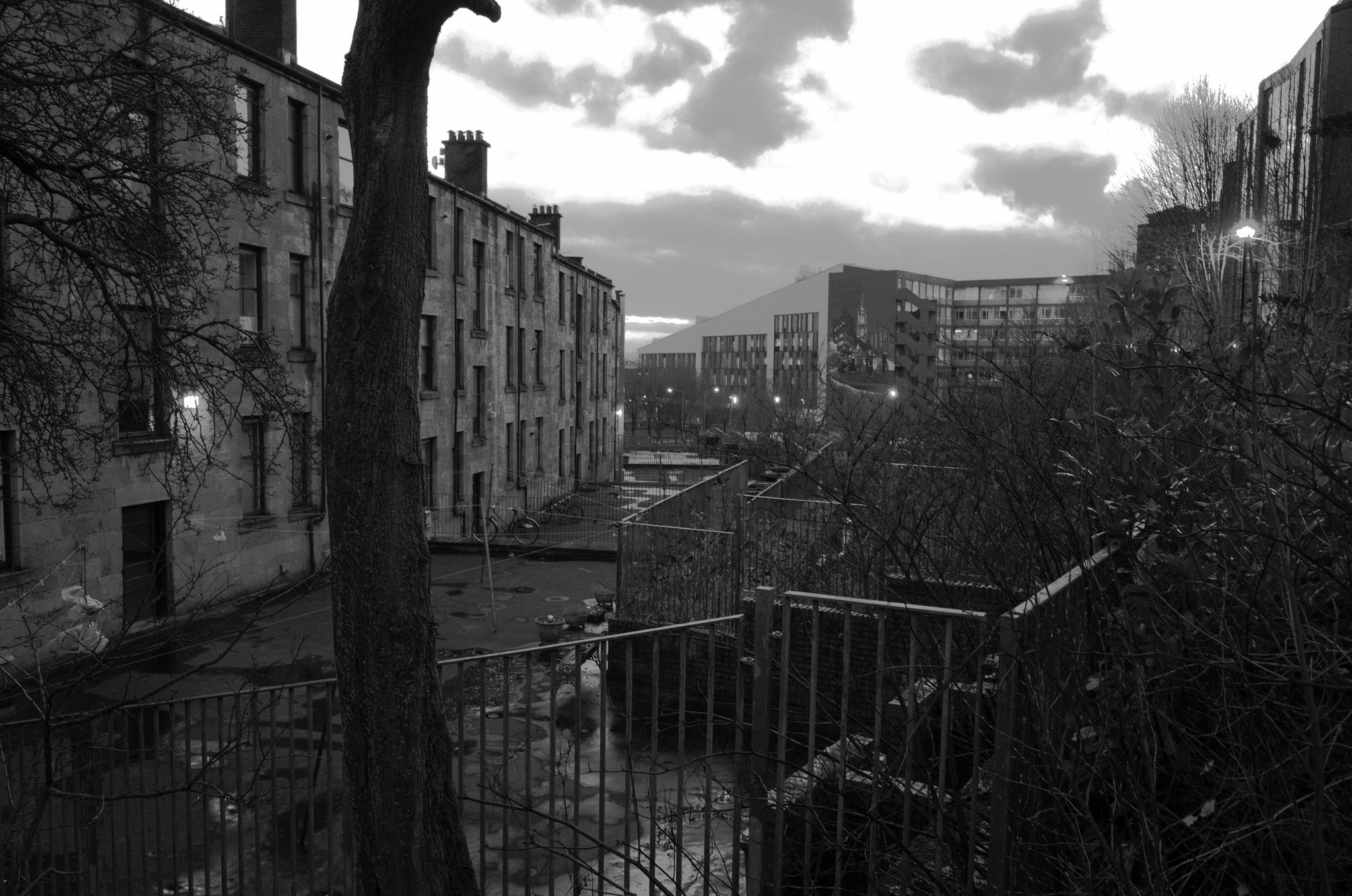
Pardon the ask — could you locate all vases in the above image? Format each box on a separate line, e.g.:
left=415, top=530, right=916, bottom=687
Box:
left=594, top=590, right=615, bottom=605
left=563, top=611, right=588, bottom=626
left=536, top=616, right=565, bottom=643
left=588, top=608, right=607, bottom=620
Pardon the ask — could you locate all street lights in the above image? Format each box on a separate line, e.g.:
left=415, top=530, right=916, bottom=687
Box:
left=667, top=388, right=684, bottom=441
left=703, top=387, right=719, bottom=438
left=730, top=395, right=737, bottom=442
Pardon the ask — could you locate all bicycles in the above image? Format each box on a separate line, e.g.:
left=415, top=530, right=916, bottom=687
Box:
left=539, top=492, right=584, bottom=526
left=471, top=503, right=540, bottom=545
